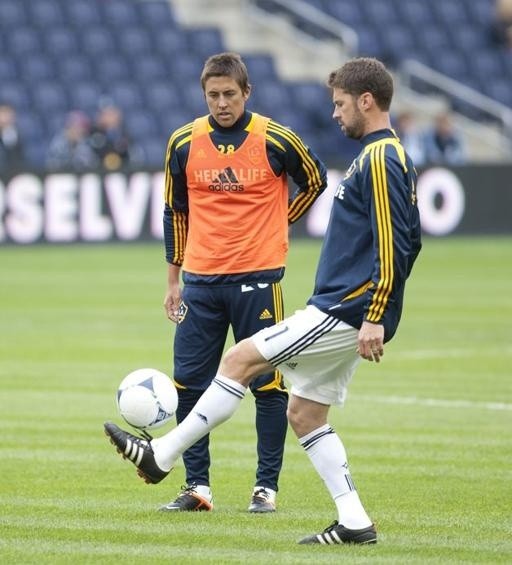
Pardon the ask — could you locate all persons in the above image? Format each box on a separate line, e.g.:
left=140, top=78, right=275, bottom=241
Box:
left=100, top=56, right=424, bottom=546
left=417, top=116, right=465, bottom=167
left=391, top=112, right=421, bottom=166
left=153, top=49, right=327, bottom=515
left=3, top=99, right=126, bottom=172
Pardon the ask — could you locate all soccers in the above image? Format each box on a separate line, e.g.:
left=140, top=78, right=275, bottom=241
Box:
left=118, top=369, right=179, bottom=430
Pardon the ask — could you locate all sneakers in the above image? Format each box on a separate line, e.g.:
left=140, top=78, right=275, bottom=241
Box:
left=298, top=520, right=377, bottom=544
left=249, top=488, right=276, bottom=513
left=104, top=423, right=174, bottom=484
left=157, top=489, right=214, bottom=512
